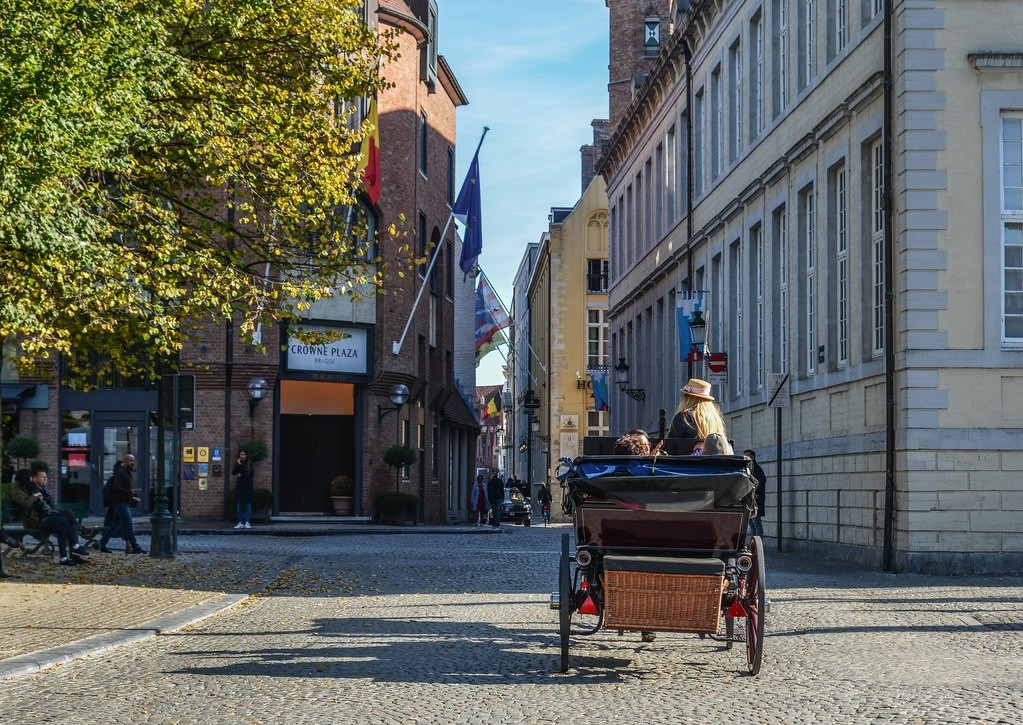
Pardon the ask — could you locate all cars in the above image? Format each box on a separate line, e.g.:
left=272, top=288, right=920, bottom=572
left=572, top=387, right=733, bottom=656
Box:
left=487, top=487, right=533, bottom=526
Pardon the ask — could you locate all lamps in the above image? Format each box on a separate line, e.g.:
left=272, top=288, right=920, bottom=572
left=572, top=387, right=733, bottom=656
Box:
left=496, top=427, right=514, bottom=447
left=246, top=378, right=268, bottom=416
left=688, top=310, right=729, bottom=372
left=379, top=385, right=411, bottom=423
left=614, top=358, right=644, bottom=405
left=530, top=415, right=549, bottom=441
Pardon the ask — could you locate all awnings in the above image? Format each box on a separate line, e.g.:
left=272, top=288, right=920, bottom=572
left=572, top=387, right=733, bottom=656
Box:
left=0, top=384, right=36, bottom=401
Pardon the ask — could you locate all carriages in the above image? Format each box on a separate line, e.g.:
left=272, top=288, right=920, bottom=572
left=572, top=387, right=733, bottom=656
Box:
left=543, top=405, right=775, bottom=677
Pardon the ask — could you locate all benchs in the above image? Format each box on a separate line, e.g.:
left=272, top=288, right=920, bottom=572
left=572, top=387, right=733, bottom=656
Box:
left=0, top=502, right=88, bottom=562
left=584, top=434, right=734, bottom=472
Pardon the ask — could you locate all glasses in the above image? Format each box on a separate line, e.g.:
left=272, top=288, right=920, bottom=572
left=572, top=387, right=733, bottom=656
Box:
left=692, top=448, right=702, bottom=456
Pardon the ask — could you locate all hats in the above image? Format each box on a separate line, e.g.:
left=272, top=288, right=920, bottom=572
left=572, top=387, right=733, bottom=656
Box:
left=700, top=433, right=735, bottom=456
left=679, top=378, right=715, bottom=401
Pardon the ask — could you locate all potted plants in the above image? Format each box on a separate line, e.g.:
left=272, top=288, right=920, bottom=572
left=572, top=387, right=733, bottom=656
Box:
left=331, top=477, right=357, bottom=515
left=226, top=443, right=277, bottom=521
left=370, top=446, right=419, bottom=523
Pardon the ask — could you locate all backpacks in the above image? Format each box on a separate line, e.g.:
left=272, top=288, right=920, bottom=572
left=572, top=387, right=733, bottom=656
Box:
left=102, top=467, right=132, bottom=507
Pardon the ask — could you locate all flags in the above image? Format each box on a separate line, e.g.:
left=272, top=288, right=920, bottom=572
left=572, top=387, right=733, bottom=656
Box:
left=484, top=390, right=501, bottom=422
left=475, top=273, right=517, bottom=368
left=356, top=55, right=381, bottom=207
left=450, top=149, right=482, bottom=282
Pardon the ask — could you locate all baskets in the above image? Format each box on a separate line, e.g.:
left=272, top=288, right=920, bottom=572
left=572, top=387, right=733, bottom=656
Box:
left=601, top=570, right=724, bottom=634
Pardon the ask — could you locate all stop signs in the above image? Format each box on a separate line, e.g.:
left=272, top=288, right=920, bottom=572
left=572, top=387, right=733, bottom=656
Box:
left=708, top=353, right=727, bottom=372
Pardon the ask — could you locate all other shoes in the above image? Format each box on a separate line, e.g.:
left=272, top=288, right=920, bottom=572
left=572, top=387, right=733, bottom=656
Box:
left=72, top=546, right=91, bottom=557
left=60, top=559, right=78, bottom=566
left=101, top=545, right=112, bottom=554
left=69, top=556, right=91, bottom=565
left=132, top=546, right=148, bottom=554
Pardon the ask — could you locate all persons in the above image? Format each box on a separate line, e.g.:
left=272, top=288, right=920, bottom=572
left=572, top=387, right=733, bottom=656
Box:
left=613, top=430, right=663, bottom=456
left=505, top=476, right=531, bottom=500
left=232, top=449, right=254, bottom=528
left=487, top=469, right=504, bottom=527
left=0, top=469, right=98, bottom=578
left=538, top=482, right=552, bottom=524
left=744, top=449, right=767, bottom=542
left=471, top=474, right=490, bottom=526
left=665, top=379, right=734, bottom=455
left=92, top=454, right=147, bottom=554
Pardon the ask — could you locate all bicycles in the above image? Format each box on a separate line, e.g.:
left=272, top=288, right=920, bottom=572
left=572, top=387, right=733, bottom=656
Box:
left=537, top=500, right=554, bottom=528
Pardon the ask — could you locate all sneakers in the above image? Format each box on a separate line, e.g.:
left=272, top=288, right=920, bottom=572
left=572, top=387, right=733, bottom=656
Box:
left=246, top=522, right=252, bottom=529
left=234, top=522, right=245, bottom=529
